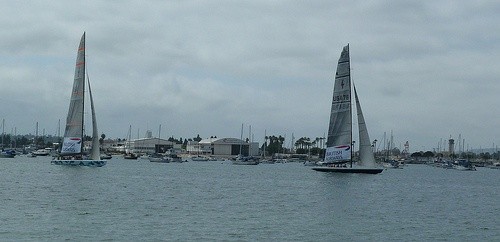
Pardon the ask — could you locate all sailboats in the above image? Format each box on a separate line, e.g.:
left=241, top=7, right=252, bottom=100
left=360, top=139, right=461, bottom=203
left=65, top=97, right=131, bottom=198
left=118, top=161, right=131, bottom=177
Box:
left=51, top=31, right=108, bottom=168
left=310, top=43, right=383, bottom=174
left=86, top=124, right=328, bottom=171
left=1, top=118, right=64, bottom=159
left=369, top=131, right=500, bottom=176
